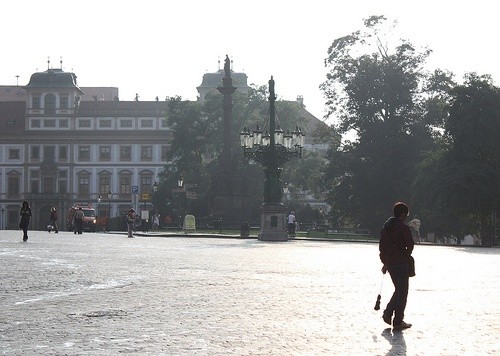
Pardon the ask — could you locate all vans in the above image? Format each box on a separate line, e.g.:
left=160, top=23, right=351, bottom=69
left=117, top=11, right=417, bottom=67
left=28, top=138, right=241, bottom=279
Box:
left=67, top=205, right=97, bottom=233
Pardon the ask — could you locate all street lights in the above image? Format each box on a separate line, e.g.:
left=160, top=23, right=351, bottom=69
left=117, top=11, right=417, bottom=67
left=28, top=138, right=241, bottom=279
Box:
left=177, top=175, right=185, bottom=231
left=238, top=75, right=306, bottom=242
left=106, top=190, right=112, bottom=230
left=97, top=196, right=102, bottom=230
left=153, top=181, right=158, bottom=231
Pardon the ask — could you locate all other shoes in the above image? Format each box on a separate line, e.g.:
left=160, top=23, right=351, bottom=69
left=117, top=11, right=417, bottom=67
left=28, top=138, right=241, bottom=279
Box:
left=394, top=322, right=412, bottom=329
left=382, top=314, right=392, bottom=325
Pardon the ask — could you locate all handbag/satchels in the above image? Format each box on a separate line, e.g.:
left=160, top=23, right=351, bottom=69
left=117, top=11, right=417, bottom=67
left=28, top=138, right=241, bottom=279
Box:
left=408, top=256, right=415, bottom=277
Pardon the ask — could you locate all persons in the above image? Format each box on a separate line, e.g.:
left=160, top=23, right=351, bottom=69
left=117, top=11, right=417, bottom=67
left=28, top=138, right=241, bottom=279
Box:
left=287, top=211, right=296, bottom=235
left=127, top=209, right=135, bottom=238
left=20, top=200, right=32, bottom=241
left=409, top=215, right=421, bottom=244
left=149, top=208, right=177, bottom=231
left=48, top=206, right=58, bottom=233
left=76, top=207, right=84, bottom=234
left=379, top=202, right=416, bottom=328
